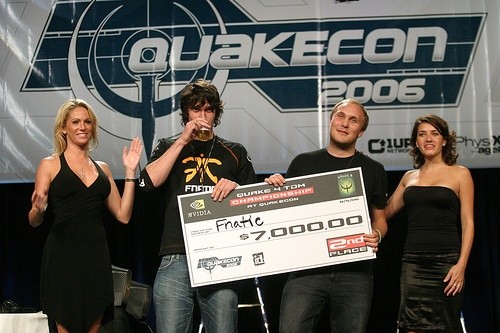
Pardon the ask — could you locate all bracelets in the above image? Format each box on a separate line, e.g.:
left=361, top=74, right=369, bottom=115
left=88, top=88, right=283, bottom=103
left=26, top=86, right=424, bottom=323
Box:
left=375, top=229, right=382, bottom=243
left=125, top=178, right=135, bottom=183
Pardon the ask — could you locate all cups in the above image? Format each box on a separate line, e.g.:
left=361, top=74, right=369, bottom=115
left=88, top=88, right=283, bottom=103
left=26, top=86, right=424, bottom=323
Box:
left=197, top=119, right=212, bottom=141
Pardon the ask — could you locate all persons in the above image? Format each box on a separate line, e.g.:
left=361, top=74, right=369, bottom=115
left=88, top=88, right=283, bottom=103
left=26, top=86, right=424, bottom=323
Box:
left=29, top=99, right=143, bottom=333
left=264, top=98, right=389, bottom=332
left=139, top=80, right=256, bottom=333
left=386, top=116, right=474, bottom=333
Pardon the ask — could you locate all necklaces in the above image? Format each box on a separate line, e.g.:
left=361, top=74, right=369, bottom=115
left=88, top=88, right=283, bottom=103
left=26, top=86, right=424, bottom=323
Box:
left=65, top=152, right=87, bottom=179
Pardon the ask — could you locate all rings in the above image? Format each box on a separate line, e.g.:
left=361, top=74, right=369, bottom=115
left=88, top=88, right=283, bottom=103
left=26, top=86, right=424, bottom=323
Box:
left=454, top=285, right=457, bottom=288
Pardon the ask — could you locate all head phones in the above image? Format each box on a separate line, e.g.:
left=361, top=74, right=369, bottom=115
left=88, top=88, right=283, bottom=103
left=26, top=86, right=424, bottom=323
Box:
left=2, top=298, right=36, bottom=313
